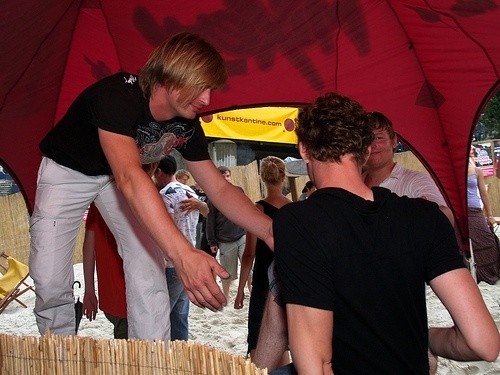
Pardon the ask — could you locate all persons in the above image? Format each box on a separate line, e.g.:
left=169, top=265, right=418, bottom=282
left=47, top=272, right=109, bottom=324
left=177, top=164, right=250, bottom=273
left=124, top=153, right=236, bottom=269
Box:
left=82, top=153, right=316, bottom=341
left=30, top=32, right=273, bottom=342
left=360, top=111, right=455, bottom=228
left=250, top=91, right=500, bottom=375
left=234, top=156, right=293, bottom=370
left=468, top=148, right=500, bottom=284
